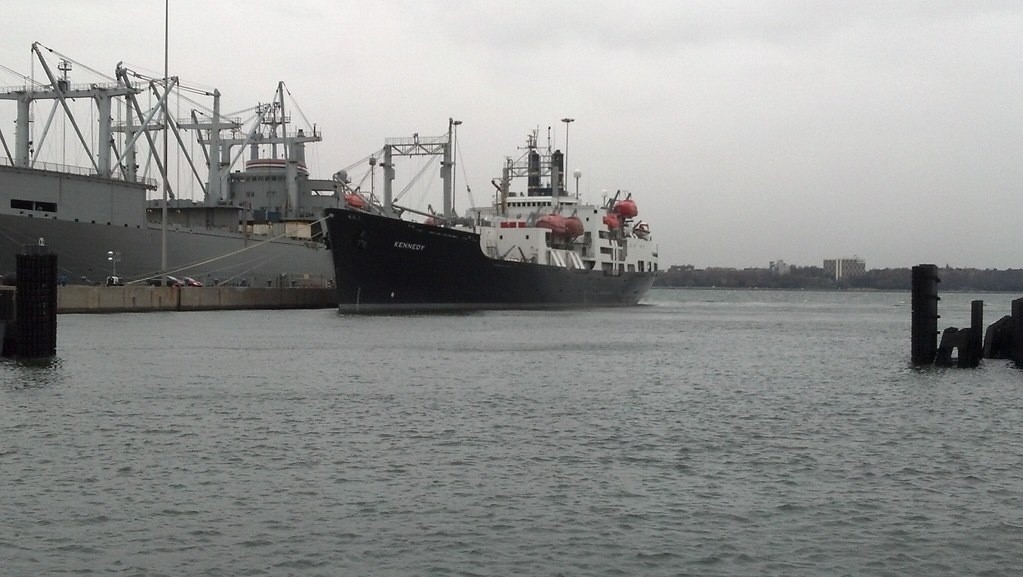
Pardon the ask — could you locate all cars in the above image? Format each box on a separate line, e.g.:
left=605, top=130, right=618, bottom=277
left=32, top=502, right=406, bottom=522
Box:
left=147, top=274, right=186, bottom=287
left=182, top=277, right=204, bottom=287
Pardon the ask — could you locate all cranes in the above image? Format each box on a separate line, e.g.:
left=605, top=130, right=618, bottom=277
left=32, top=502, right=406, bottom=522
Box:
left=0, top=42, right=323, bottom=203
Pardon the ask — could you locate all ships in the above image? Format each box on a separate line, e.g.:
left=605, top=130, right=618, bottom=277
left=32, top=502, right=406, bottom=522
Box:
left=320, top=125, right=660, bottom=315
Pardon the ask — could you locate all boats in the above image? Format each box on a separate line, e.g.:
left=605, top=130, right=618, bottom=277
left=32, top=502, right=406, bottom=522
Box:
left=535, top=213, right=567, bottom=234
left=633, top=222, right=651, bottom=238
left=566, top=216, right=584, bottom=236
left=613, top=198, right=639, bottom=218
left=345, top=195, right=366, bottom=207
left=604, top=214, right=620, bottom=228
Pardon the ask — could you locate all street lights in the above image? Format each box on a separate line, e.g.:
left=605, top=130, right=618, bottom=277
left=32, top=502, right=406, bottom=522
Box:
left=560, top=118, right=574, bottom=190
left=107, top=250, right=116, bottom=276
left=451, top=120, right=463, bottom=213
